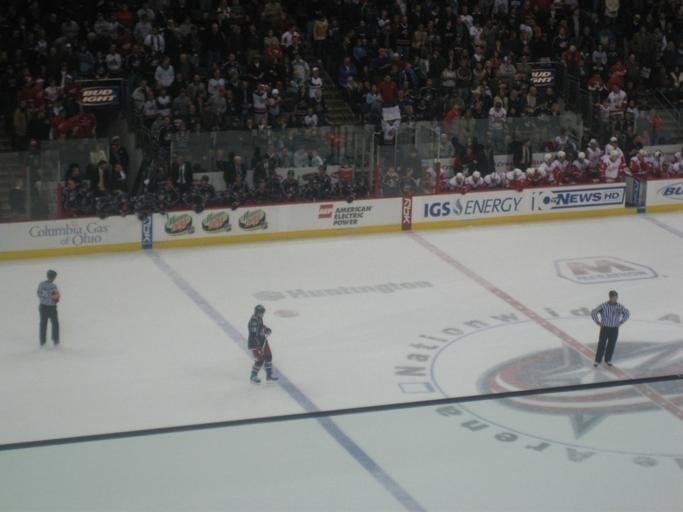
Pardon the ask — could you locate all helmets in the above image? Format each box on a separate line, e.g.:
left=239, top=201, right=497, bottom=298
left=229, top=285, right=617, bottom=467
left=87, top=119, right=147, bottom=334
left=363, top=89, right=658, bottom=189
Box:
left=255, top=305, right=265, bottom=316
left=639, top=149, right=681, bottom=172
left=47, top=271, right=56, bottom=279
left=456, top=137, right=619, bottom=182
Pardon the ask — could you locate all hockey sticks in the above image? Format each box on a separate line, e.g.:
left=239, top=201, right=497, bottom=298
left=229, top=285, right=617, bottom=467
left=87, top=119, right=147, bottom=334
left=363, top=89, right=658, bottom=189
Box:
left=252, top=333, right=269, bottom=361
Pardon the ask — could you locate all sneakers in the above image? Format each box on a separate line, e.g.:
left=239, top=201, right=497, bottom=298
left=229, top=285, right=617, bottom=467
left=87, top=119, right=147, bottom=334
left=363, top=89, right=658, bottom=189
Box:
left=594, top=361, right=600, bottom=367
left=266, top=376, right=279, bottom=381
left=605, top=360, right=612, bottom=366
left=250, top=376, right=260, bottom=383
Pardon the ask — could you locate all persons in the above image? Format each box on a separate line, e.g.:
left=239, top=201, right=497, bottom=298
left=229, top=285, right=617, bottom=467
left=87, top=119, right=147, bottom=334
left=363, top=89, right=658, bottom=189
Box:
left=590, top=289, right=630, bottom=368
left=1, top=0, right=682, bottom=221
left=36, top=269, right=62, bottom=348
left=245, top=303, right=280, bottom=383
